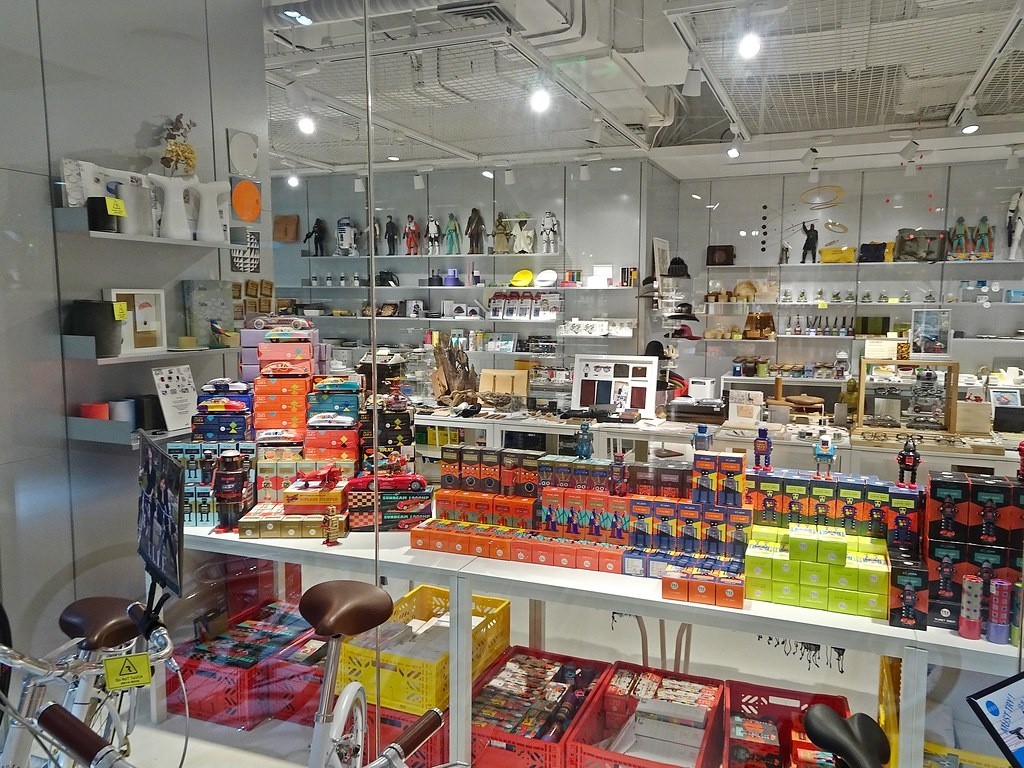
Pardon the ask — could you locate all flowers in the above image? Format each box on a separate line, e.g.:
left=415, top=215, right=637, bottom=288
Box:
left=155, top=112, right=197, bottom=179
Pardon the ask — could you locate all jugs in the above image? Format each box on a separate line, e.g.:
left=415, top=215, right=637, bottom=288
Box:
left=998, top=366, right=1024, bottom=384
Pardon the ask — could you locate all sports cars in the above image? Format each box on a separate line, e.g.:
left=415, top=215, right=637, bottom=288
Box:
left=349, top=472, right=427, bottom=491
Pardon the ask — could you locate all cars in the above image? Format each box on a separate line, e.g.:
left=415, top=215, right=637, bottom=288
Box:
left=260, top=360, right=309, bottom=378
left=194, top=396, right=248, bottom=415
left=305, top=411, right=356, bottom=431
left=263, top=326, right=313, bottom=342
left=199, top=377, right=252, bottom=395
left=315, top=377, right=362, bottom=394
left=255, top=428, right=302, bottom=446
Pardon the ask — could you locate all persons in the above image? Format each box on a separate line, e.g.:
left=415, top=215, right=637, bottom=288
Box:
left=800, top=221, right=818, bottom=264
left=403, top=215, right=420, bottom=255
left=539, top=209, right=559, bottom=254
left=492, top=212, right=510, bottom=254
left=442, top=213, right=461, bottom=255
left=360, top=216, right=381, bottom=256
left=949, top=216, right=971, bottom=253
left=424, top=215, right=441, bottom=255
left=385, top=215, right=397, bottom=256
left=138, top=445, right=177, bottom=577
left=1006, top=188, right=1024, bottom=261
left=464, top=208, right=486, bottom=254
left=303, top=218, right=326, bottom=257
left=511, top=211, right=536, bottom=254
left=972, top=216, right=994, bottom=253
left=838, top=379, right=867, bottom=417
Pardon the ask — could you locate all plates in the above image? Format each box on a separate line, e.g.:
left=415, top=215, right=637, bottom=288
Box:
left=509, top=269, right=533, bottom=287
left=167, top=346, right=210, bottom=351
left=536, top=269, right=557, bottom=287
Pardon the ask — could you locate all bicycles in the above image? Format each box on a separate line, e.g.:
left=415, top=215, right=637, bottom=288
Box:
left=0, top=592, right=191, bottom=768
left=299, top=580, right=472, bottom=768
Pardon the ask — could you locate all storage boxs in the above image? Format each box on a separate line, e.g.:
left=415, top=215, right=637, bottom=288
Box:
left=102, top=289, right=166, bottom=355
left=167, top=327, right=1024, bottom=632
left=164, top=585, right=1014, bottom=768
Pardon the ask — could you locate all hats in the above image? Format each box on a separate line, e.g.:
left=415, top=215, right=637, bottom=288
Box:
left=639, top=276, right=658, bottom=296
left=668, top=258, right=688, bottom=277
left=646, top=341, right=671, bottom=359
left=668, top=303, right=699, bottom=323
left=664, top=324, right=702, bottom=340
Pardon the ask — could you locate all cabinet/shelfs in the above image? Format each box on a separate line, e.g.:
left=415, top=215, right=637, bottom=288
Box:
left=53, top=207, right=244, bottom=451
left=273, top=251, right=1024, bottom=358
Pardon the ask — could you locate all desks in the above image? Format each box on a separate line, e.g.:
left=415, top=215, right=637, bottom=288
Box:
left=852, top=373, right=986, bottom=402
left=712, top=428, right=851, bottom=475
left=987, top=385, right=1024, bottom=402
left=493, top=412, right=602, bottom=458
left=598, top=423, right=723, bottom=461
left=720, top=371, right=852, bottom=400
left=144, top=527, right=478, bottom=768
left=456, top=556, right=917, bottom=768
left=1005, top=449, right=1021, bottom=477
left=914, top=626, right=1024, bottom=768
left=852, top=445, right=1006, bottom=485
left=412, top=405, right=513, bottom=446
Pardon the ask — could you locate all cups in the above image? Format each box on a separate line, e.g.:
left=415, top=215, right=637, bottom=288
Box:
left=732, top=355, right=770, bottom=377
left=177, top=336, right=199, bottom=349
left=707, top=291, right=754, bottom=303
left=704, top=329, right=731, bottom=339
left=78, top=398, right=136, bottom=433
left=86, top=196, right=117, bottom=234
left=833, top=403, right=848, bottom=426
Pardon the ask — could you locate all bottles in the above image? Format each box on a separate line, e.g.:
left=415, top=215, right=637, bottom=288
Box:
left=959, top=280, right=970, bottom=303
left=976, top=280, right=988, bottom=303
left=784, top=327, right=854, bottom=336
left=468, top=330, right=483, bottom=351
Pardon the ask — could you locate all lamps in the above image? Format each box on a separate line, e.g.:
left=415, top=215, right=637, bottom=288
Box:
left=584, top=107, right=602, bottom=144
left=800, top=136, right=832, bottom=168
left=1005, top=145, right=1020, bottom=169
left=961, top=95, right=980, bottom=135
left=413, top=165, right=434, bottom=190
left=725, top=123, right=743, bottom=158
left=355, top=170, right=368, bottom=192
left=495, top=160, right=515, bottom=185
left=579, top=152, right=602, bottom=181
left=901, top=141, right=932, bottom=177
left=682, top=51, right=703, bottom=97
left=808, top=157, right=833, bottom=182
left=286, top=61, right=318, bottom=135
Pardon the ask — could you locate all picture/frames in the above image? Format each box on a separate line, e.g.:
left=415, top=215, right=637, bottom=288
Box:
left=989, top=389, right=1022, bottom=419
left=653, top=236, right=673, bottom=308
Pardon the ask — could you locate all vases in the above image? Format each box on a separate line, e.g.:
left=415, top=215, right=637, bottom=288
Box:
left=148, top=172, right=199, bottom=240
left=190, top=180, right=231, bottom=243
